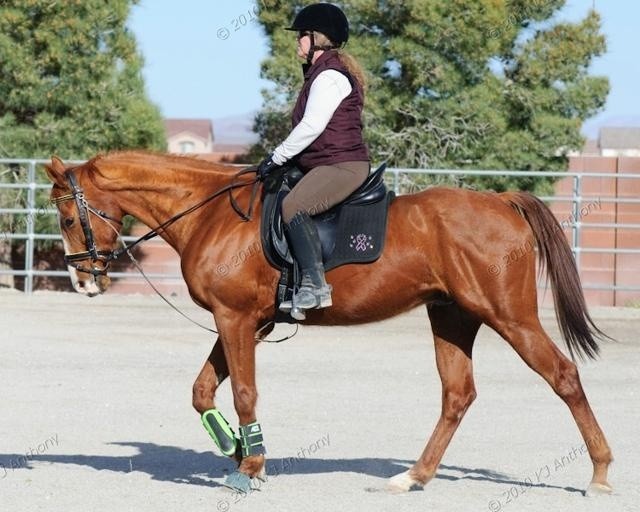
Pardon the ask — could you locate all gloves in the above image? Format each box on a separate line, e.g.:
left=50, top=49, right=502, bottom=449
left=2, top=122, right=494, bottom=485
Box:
left=256, top=158, right=278, bottom=182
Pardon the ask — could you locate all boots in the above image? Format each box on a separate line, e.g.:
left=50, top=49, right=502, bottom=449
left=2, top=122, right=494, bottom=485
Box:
left=278, top=211, right=334, bottom=313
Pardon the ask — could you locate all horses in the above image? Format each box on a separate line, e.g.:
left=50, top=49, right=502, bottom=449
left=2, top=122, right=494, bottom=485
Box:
left=41, top=146, right=617, bottom=500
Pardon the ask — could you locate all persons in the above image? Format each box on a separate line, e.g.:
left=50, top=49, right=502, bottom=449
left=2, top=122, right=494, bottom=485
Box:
left=252, top=3, right=369, bottom=314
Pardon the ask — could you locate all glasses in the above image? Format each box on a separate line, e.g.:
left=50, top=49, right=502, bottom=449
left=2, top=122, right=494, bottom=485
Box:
left=299, top=32, right=314, bottom=39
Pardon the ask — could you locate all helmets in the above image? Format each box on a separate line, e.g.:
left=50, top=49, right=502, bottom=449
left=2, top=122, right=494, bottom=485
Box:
left=283, top=2, right=350, bottom=44
